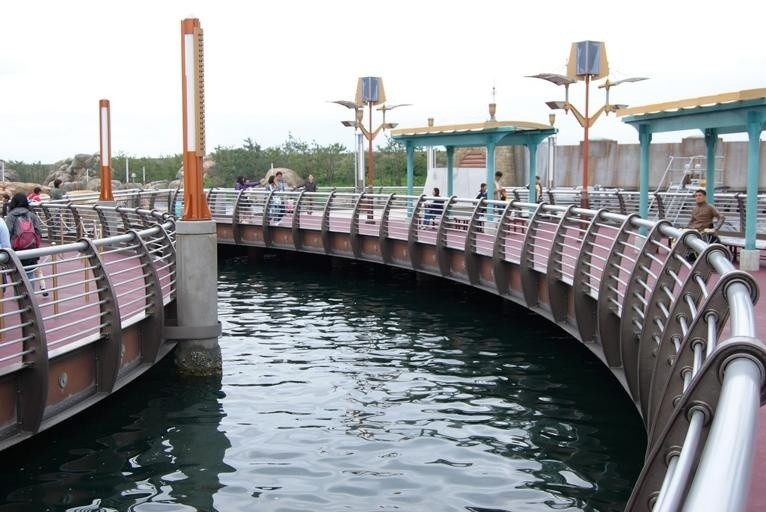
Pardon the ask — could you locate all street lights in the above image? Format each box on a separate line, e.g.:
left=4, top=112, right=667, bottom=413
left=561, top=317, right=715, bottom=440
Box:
left=97, top=99, right=115, bottom=201
left=330, top=99, right=415, bottom=225
left=354, top=132, right=366, bottom=194
left=178, top=16, right=215, bottom=221
left=524, top=73, right=651, bottom=246
left=130, top=172, right=136, bottom=183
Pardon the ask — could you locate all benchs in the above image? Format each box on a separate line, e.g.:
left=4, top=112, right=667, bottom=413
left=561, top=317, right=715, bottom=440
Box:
left=668, top=227, right=766, bottom=267
left=419, top=215, right=526, bottom=235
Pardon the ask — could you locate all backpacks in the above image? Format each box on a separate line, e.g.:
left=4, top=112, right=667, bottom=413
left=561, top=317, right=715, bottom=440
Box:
left=10, top=215, right=41, bottom=251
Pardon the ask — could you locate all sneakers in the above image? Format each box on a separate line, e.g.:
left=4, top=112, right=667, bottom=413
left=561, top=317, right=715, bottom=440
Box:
left=41, top=288, right=49, bottom=297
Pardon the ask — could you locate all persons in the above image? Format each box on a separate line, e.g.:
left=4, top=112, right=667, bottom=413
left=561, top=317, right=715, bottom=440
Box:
left=685, top=189, right=725, bottom=261
left=233, top=171, right=317, bottom=222
left=526, top=176, right=542, bottom=227
left=49, top=179, right=66, bottom=230
left=417, top=189, right=443, bottom=230
left=492, top=171, right=507, bottom=230
left=0, top=218, right=11, bottom=296
left=0, top=190, right=12, bottom=221
left=474, top=183, right=487, bottom=233
left=27, top=187, right=42, bottom=202
left=0, top=193, right=48, bottom=302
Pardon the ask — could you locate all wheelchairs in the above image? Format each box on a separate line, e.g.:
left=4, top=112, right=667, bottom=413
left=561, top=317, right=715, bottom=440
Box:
left=669, top=224, right=722, bottom=265
